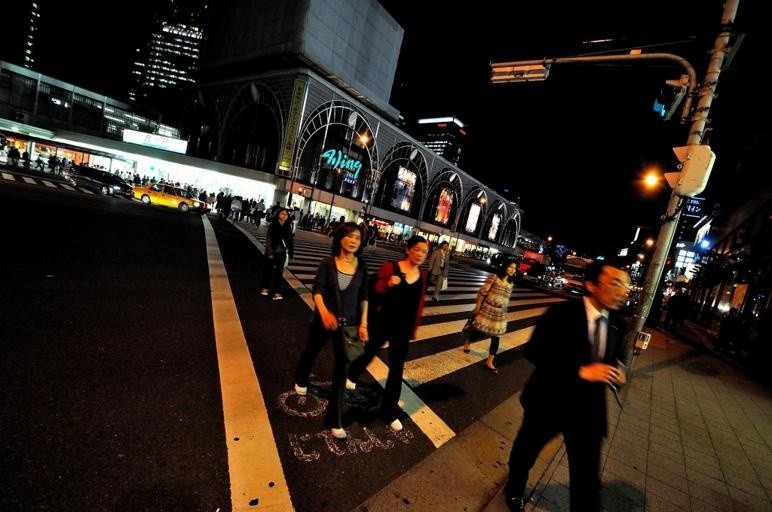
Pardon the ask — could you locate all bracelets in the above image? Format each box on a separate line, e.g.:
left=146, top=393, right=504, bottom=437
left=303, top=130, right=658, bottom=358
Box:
left=358, top=321, right=369, bottom=329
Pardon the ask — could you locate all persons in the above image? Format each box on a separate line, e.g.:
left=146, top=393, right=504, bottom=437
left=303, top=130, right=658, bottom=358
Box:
left=363, top=223, right=488, bottom=260
left=649, top=281, right=754, bottom=363
left=260, top=208, right=293, bottom=300
left=266, top=202, right=344, bottom=234
left=504, top=258, right=634, bottom=512
left=2, top=140, right=67, bottom=175
left=346, top=235, right=429, bottom=433
left=200, top=192, right=265, bottom=228
left=78, top=163, right=197, bottom=198
left=292, top=222, right=371, bottom=438
left=429, top=241, right=448, bottom=301
left=464, top=258, right=516, bottom=375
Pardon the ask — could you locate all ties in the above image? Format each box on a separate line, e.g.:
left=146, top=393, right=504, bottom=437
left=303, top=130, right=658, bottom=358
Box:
left=594, top=318, right=602, bottom=357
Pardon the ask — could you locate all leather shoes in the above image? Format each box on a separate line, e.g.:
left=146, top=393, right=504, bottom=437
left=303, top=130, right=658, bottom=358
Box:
left=464, top=343, right=470, bottom=353
left=488, top=364, right=498, bottom=374
left=506, top=491, right=526, bottom=511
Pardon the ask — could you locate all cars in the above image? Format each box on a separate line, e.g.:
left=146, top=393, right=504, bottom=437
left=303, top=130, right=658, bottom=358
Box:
left=132, top=181, right=211, bottom=213
left=66, top=166, right=133, bottom=198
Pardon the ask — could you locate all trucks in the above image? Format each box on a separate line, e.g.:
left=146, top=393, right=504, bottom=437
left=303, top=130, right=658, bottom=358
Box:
left=563, top=255, right=592, bottom=292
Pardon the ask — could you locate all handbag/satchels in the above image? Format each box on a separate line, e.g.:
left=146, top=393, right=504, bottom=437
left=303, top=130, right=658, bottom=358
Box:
left=340, top=325, right=367, bottom=361
left=441, top=277, right=448, bottom=289
left=468, top=312, right=475, bottom=322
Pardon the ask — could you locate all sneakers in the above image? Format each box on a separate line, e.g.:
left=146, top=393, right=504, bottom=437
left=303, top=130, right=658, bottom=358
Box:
left=331, top=428, right=347, bottom=438
left=346, top=378, right=356, bottom=390
left=295, top=384, right=306, bottom=395
left=273, top=294, right=283, bottom=300
left=261, top=289, right=268, bottom=295
left=389, top=419, right=402, bottom=431
